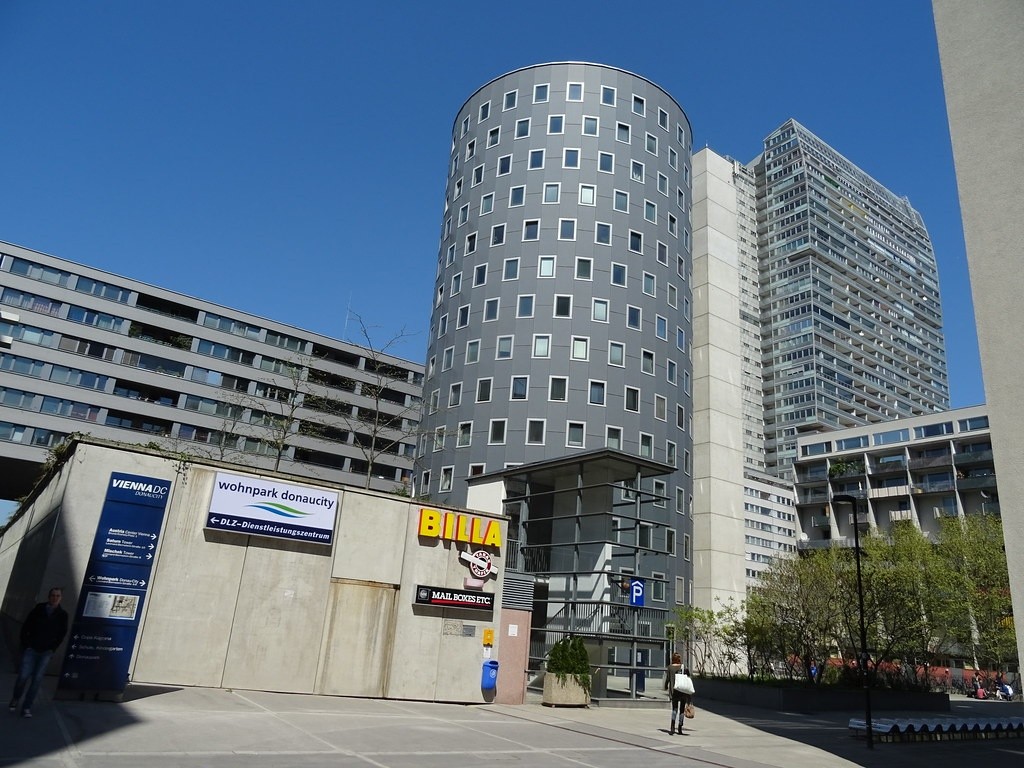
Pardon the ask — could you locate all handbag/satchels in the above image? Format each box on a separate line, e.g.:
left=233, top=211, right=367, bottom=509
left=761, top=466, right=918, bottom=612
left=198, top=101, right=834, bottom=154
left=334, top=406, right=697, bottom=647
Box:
left=673, top=663, right=695, bottom=695
left=683, top=701, right=696, bottom=718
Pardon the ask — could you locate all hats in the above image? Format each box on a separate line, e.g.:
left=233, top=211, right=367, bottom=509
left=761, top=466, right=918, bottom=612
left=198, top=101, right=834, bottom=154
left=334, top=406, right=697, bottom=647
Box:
left=945, top=668, right=949, bottom=672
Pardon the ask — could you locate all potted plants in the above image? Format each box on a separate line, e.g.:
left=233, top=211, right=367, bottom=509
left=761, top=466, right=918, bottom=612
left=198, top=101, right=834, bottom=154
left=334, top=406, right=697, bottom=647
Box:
left=542, top=637, right=592, bottom=708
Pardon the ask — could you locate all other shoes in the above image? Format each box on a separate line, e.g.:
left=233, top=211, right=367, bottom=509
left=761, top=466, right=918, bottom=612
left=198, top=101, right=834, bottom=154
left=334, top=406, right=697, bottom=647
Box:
left=8, top=700, right=16, bottom=711
left=22, top=706, right=33, bottom=718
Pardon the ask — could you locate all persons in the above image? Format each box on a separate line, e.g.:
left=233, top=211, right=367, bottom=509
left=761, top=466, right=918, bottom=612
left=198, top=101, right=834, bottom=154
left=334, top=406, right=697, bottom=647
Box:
left=9, top=588, right=68, bottom=718
left=972, top=672, right=1013, bottom=701
left=665, top=653, right=691, bottom=735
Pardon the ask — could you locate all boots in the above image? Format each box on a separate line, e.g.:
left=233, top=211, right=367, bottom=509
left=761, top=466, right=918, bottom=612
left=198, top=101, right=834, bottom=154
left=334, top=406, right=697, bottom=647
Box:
left=677, top=719, right=684, bottom=735
left=669, top=719, right=675, bottom=735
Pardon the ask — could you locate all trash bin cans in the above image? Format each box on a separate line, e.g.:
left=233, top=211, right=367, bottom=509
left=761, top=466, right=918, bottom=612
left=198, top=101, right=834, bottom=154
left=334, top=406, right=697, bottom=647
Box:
left=481, top=661, right=499, bottom=690
